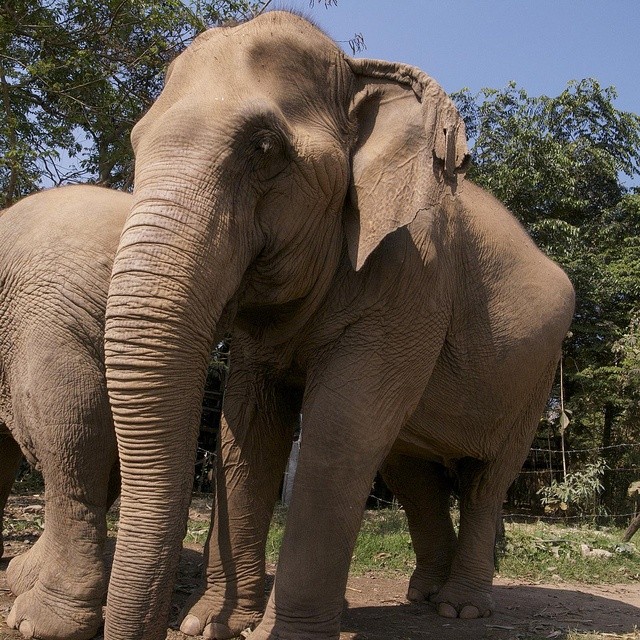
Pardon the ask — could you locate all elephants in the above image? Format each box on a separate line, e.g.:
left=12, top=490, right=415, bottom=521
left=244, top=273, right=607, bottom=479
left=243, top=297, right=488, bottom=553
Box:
left=0, top=182, right=134, bottom=640
left=104, top=9, right=577, bottom=639
